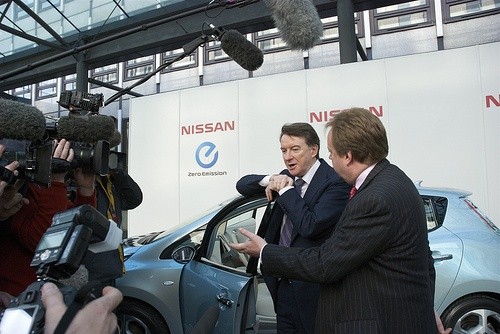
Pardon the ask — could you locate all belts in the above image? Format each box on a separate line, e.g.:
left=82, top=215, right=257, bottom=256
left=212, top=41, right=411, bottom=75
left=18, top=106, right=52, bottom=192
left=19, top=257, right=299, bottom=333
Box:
left=282, top=278, right=296, bottom=284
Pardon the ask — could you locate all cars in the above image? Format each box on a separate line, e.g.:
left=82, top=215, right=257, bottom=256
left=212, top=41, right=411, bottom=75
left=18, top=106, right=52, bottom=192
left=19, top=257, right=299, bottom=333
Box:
left=111, top=178, right=500, bottom=334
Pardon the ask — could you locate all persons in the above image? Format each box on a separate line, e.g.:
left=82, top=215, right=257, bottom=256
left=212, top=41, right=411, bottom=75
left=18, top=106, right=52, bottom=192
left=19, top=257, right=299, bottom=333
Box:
left=228, top=107, right=452, bottom=334
left=236, top=122, right=354, bottom=334
left=0, top=133, right=143, bottom=334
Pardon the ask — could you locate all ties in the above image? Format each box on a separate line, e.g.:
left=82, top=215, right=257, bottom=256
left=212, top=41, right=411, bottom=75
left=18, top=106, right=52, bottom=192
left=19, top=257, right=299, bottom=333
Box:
left=350, top=187, right=356, bottom=199
left=279, top=180, right=306, bottom=248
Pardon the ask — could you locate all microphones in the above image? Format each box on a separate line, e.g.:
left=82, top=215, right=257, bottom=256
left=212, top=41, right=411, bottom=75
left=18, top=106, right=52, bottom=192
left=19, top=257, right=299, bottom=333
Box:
left=206, top=22, right=264, bottom=72
left=193, top=305, right=220, bottom=334
left=263, top=0, right=324, bottom=51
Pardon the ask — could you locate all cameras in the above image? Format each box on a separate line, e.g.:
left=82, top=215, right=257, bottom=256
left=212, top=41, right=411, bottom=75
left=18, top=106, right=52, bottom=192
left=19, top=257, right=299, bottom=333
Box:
left=0, top=205, right=126, bottom=334
